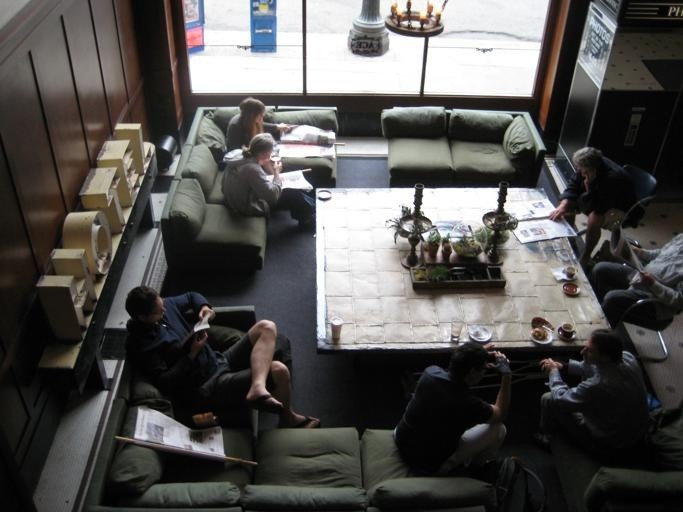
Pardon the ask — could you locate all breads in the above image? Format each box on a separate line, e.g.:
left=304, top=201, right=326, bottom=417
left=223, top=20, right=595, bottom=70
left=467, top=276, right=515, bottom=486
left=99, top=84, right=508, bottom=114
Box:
left=531, top=327, right=546, bottom=341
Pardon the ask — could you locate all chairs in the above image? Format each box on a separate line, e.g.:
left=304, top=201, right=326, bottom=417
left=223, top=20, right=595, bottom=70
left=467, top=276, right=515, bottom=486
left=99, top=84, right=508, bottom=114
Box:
left=570, top=163, right=682, bottom=362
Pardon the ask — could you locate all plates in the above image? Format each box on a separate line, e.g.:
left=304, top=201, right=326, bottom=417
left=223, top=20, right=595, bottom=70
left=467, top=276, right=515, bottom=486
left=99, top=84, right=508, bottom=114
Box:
left=469, top=326, right=493, bottom=342
left=562, top=283, right=581, bottom=296
left=531, top=317, right=555, bottom=333
left=558, top=327, right=576, bottom=341
left=529, top=329, right=553, bottom=345
left=318, top=190, right=331, bottom=200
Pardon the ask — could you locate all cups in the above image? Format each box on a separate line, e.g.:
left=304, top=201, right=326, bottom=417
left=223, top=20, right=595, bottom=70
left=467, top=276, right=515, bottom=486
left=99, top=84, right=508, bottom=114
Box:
left=561, top=266, right=576, bottom=278
left=270, top=156, right=280, bottom=168
left=562, top=323, right=577, bottom=338
left=451, top=316, right=464, bottom=340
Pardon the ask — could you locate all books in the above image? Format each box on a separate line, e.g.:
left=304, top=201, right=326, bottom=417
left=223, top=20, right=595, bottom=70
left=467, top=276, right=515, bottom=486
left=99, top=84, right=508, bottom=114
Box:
left=175, top=314, right=211, bottom=346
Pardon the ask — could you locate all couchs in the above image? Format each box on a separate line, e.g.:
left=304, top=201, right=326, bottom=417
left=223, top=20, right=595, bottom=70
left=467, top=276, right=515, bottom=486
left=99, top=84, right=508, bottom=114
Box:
left=158, top=104, right=338, bottom=276
left=380, top=105, right=548, bottom=189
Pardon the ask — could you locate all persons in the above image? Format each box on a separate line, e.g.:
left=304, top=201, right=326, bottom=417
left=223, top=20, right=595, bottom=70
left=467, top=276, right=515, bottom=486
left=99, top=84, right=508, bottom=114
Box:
left=224, top=96, right=291, bottom=174
left=219, top=132, right=315, bottom=226
left=590, top=232, right=682, bottom=328
left=124, top=285, right=321, bottom=428
left=530, top=328, right=650, bottom=448
left=548, top=144, right=632, bottom=266
left=390, top=341, right=511, bottom=474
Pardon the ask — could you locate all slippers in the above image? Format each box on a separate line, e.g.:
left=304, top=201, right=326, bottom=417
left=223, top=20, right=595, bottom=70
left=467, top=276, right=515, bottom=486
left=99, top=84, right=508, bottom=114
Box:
left=281, top=415, right=319, bottom=428
left=249, top=393, right=283, bottom=414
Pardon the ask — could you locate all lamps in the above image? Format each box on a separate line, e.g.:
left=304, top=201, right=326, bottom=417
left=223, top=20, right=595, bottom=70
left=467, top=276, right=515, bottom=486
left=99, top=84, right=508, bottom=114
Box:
left=386, top=0, right=452, bottom=37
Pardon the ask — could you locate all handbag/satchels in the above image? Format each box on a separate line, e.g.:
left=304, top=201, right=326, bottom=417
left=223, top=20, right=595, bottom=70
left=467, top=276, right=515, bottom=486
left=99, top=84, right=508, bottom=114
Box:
left=478, top=456, right=546, bottom=511
